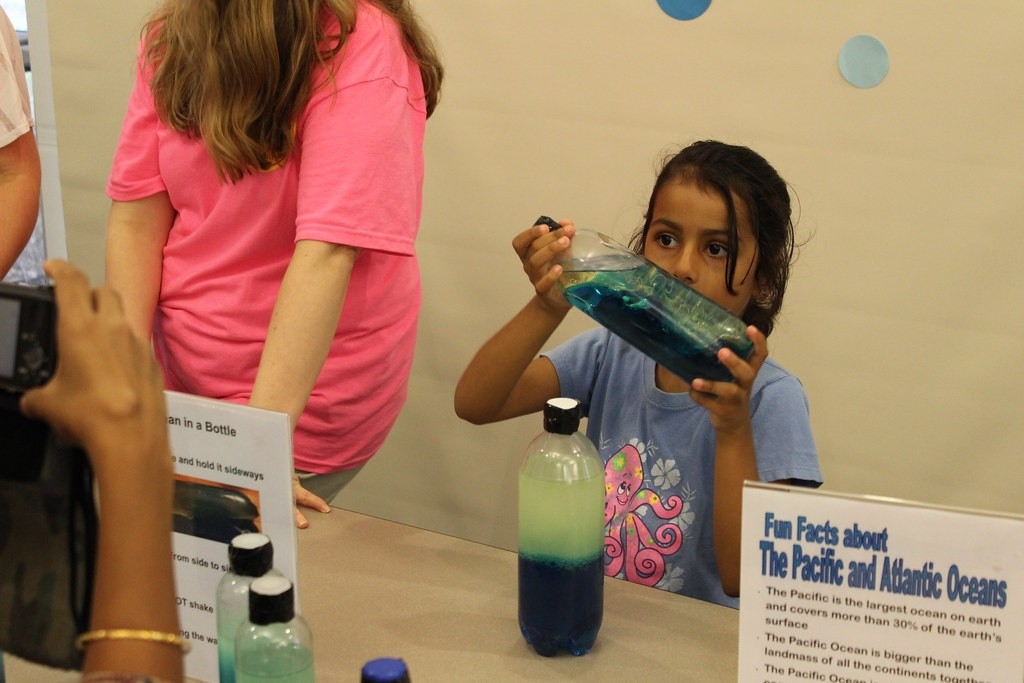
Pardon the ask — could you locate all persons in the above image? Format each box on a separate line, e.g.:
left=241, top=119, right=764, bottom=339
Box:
left=0, top=253, right=192, bottom=683
left=103, top=1, right=446, bottom=527
left=0, top=4, right=42, bottom=281
left=455, top=139, right=823, bottom=612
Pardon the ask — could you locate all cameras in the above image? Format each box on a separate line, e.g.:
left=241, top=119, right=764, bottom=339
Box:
left=0, top=283, right=58, bottom=392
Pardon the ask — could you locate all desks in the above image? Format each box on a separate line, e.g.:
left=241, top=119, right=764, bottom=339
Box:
left=1, top=501, right=739, bottom=683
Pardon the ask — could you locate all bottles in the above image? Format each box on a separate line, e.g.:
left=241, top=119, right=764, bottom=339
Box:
left=235, top=574, right=316, bottom=683
left=515, top=398, right=608, bottom=657
left=530, top=215, right=755, bottom=396
left=216, top=533, right=290, bottom=682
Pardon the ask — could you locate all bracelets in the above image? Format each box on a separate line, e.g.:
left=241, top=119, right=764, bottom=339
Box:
left=73, top=625, right=190, bottom=683
